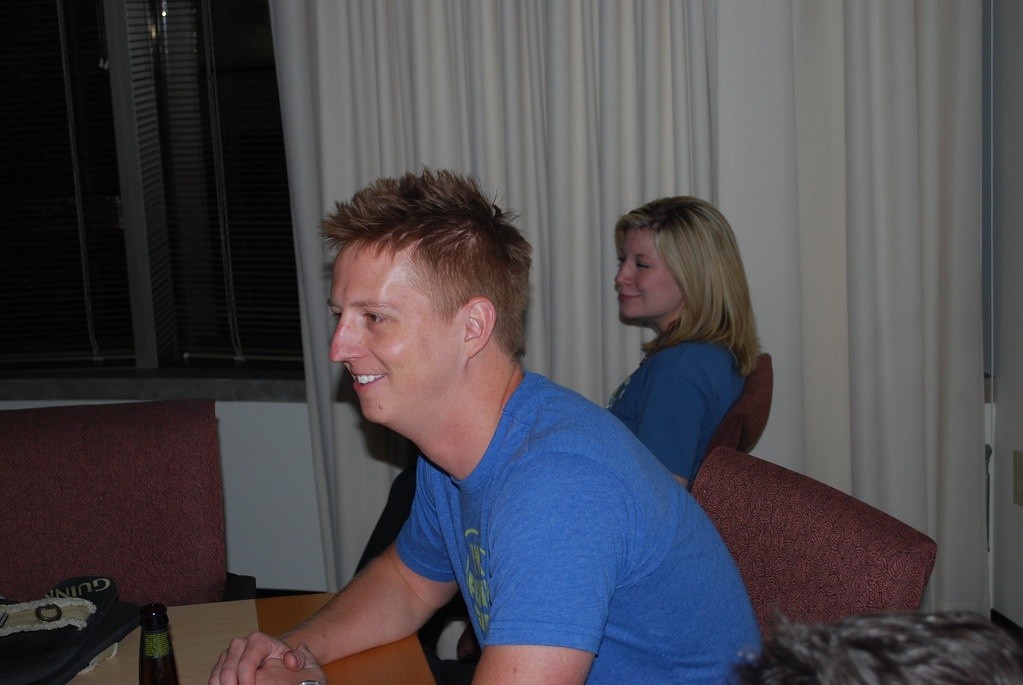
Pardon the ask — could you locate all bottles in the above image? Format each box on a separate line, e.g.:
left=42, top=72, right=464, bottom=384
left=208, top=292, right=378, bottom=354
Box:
left=139, top=602, right=180, bottom=685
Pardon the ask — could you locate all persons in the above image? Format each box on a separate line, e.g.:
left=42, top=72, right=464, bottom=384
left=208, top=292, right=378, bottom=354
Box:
left=207, top=163, right=771, bottom=685
left=732, top=611, right=1023, bottom=685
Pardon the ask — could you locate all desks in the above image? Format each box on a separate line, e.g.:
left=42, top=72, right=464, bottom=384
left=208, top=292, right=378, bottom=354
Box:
left=65, top=590, right=438, bottom=685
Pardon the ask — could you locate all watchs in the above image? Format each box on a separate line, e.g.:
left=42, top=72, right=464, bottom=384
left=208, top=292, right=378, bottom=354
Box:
left=298, top=681, right=320, bottom=685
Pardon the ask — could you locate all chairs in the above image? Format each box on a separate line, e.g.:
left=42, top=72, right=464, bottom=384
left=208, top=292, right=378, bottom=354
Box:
left=689, top=445, right=938, bottom=640
left=0, top=398, right=226, bottom=606
left=704, top=353, right=773, bottom=452
